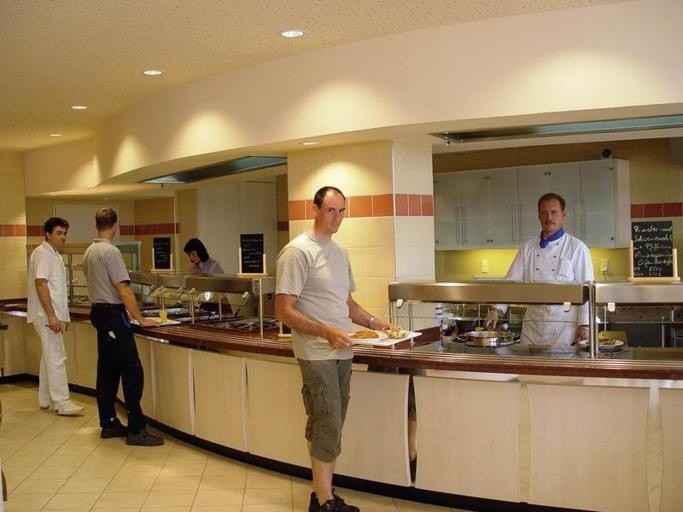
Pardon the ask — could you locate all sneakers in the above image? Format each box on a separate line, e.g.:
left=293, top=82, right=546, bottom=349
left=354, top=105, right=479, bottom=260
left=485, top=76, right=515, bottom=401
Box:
left=40, top=400, right=85, bottom=415
left=126, top=430, right=163, bottom=446
left=308, top=488, right=360, bottom=511
left=101, top=417, right=128, bottom=439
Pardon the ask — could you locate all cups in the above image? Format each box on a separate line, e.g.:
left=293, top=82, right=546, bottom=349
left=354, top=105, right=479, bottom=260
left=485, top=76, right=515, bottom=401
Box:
left=158, top=309, right=167, bottom=322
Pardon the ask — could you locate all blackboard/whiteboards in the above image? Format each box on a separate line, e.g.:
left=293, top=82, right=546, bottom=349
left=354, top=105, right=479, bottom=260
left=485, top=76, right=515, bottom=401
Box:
left=630, top=221, right=676, bottom=280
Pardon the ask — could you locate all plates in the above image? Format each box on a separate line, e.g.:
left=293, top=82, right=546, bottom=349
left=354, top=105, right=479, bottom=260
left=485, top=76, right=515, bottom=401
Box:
left=348, top=329, right=387, bottom=341
left=578, top=338, right=624, bottom=349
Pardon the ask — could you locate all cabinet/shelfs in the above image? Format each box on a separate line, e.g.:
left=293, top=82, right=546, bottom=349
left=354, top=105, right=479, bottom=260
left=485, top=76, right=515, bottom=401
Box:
left=435, top=157, right=632, bottom=252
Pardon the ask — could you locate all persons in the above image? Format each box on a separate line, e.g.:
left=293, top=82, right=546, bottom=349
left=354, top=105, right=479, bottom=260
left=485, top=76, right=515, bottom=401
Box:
left=184, top=238, right=234, bottom=316
left=27, top=216, right=85, bottom=416
left=273, top=185, right=403, bottom=511
left=83, top=207, right=165, bottom=447
left=482, top=193, right=595, bottom=348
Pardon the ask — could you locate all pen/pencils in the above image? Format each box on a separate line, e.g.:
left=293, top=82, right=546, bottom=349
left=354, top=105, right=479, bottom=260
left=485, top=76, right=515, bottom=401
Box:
left=45, top=324, right=50, bottom=327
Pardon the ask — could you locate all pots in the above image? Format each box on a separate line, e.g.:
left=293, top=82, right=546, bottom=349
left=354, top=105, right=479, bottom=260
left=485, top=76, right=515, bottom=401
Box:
left=455, top=331, right=514, bottom=346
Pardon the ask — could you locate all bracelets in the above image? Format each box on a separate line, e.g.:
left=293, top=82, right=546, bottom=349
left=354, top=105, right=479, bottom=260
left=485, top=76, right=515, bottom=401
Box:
left=369, top=316, right=376, bottom=330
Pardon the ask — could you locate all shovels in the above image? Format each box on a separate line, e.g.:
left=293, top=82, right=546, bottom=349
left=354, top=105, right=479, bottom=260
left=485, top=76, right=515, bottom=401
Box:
left=570, top=338, right=581, bottom=346
